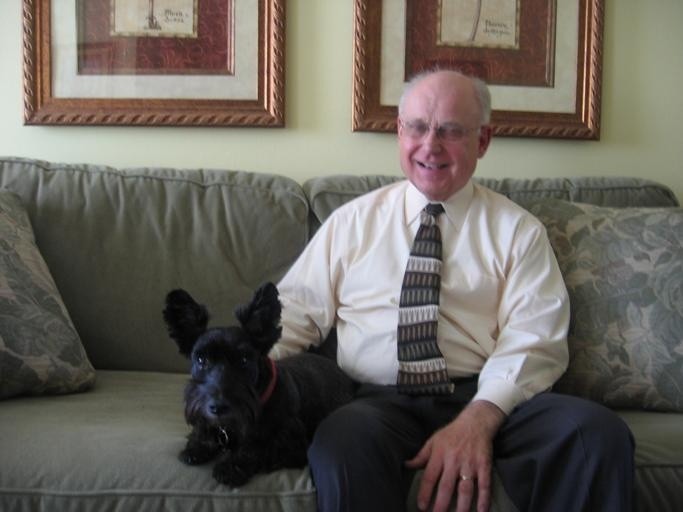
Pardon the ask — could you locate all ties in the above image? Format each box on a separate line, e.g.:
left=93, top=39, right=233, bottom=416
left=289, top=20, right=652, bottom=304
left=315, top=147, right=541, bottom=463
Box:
left=395, top=202, right=456, bottom=399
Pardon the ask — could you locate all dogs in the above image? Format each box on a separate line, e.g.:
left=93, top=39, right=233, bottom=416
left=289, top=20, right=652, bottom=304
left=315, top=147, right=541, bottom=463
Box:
left=161, top=281, right=358, bottom=489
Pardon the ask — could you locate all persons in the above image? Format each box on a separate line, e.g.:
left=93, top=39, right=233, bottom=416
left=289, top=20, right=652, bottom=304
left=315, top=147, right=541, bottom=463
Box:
left=266, top=66, right=640, bottom=512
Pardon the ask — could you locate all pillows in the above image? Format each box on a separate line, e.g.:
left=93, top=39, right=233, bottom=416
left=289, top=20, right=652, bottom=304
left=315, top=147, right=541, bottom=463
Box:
left=0, top=188, right=98, bottom=399
left=512, top=196, right=683, bottom=415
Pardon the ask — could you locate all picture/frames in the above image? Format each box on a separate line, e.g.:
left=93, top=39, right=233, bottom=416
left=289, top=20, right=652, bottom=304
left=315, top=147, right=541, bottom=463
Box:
left=349, top=0, right=604, bottom=142
left=18, top=0, right=286, bottom=132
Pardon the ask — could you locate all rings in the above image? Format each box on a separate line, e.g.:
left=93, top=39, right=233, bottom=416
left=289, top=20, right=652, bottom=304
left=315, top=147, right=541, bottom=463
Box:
left=458, top=475, right=472, bottom=481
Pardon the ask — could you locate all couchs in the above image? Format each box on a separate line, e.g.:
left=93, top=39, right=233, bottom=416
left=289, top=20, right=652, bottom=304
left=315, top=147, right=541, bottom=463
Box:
left=0, top=155, right=683, bottom=510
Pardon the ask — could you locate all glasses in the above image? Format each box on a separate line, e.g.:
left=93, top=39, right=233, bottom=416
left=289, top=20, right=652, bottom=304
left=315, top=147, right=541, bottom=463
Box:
left=400, top=118, right=483, bottom=143
